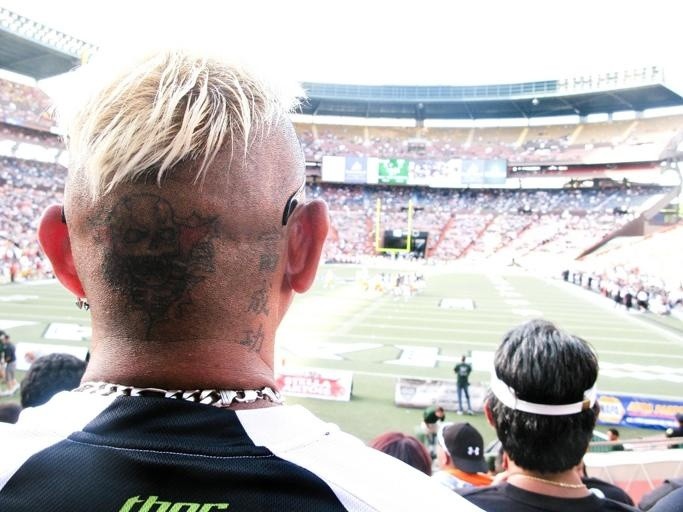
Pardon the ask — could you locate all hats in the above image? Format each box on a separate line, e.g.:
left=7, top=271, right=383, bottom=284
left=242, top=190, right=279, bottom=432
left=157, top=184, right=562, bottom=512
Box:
left=437, top=421, right=489, bottom=473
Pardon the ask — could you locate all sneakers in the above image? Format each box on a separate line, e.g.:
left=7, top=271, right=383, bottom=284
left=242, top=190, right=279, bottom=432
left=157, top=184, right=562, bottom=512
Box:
left=0, top=383, right=20, bottom=397
left=457, top=410, right=473, bottom=415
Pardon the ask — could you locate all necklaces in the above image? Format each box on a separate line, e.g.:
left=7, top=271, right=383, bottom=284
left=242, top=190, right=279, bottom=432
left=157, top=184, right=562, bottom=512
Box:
left=69, top=381, right=287, bottom=414
left=507, top=472, right=587, bottom=491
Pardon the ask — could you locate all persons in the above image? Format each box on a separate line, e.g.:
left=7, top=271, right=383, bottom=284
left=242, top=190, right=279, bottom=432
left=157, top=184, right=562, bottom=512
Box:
left=484, top=453, right=497, bottom=478
left=370, top=424, right=432, bottom=481
left=492, top=445, right=510, bottom=476
left=606, top=427, right=625, bottom=453
left=460, top=316, right=636, bottom=512
left=663, top=412, right=682, bottom=449
left=451, top=354, right=475, bottom=417
left=0, top=334, right=17, bottom=391
left=420, top=405, right=446, bottom=453
left=0, top=401, right=20, bottom=425
left=19, top=348, right=92, bottom=414
left=0, top=49, right=489, bottom=512
left=0, top=330, right=7, bottom=381
left=435, top=418, right=499, bottom=492
